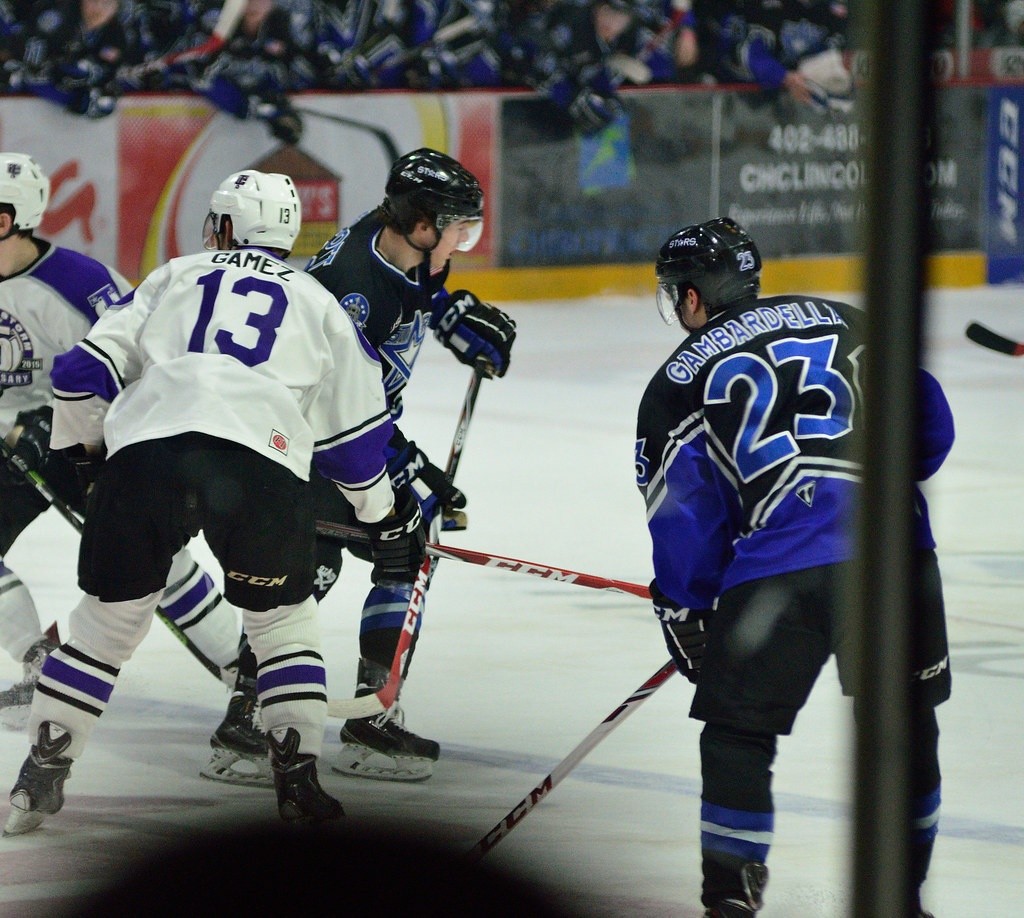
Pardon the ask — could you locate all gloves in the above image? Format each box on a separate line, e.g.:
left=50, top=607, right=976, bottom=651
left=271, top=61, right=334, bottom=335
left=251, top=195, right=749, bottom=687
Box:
left=649, top=577, right=717, bottom=685
left=6, top=414, right=52, bottom=484
left=551, top=77, right=616, bottom=141
left=429, top=288, right=517, bottom=383
left=364, top=485, right=426, bottom=586
left=388, top=441, right=467, bottom=539
left=40, top=445, right=94, bottom=515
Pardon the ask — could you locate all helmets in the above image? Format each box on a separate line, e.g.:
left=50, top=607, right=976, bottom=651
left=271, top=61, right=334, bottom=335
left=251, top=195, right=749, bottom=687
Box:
left=386, top=147, right=486, bottom=253
left=201, top=168, right=301, bottom=253
left=653, top=218, right=762, bottom=328
left=2, top=151, right=51, bottom=232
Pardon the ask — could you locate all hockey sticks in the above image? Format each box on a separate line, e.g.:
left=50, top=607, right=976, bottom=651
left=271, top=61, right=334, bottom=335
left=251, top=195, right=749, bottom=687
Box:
left=1, top=439, right=237, bottom=689
left=127, top=0, right=249, bottom=77
left=326, top=353, right=490, bottom=720
left=963, top=321, right=1024, bottom=356
left=312, top=517, right=651, bottom=601
left=611, top=0, right=692, bottom=88
left=291, top=106, right=400, bottom=163
left=468, top=658, right=679, bottom=869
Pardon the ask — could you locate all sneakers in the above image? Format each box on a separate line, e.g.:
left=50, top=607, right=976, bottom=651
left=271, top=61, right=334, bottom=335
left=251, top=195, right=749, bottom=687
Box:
left=0, top=720, right=75, bottom=841
left=264, top=726, right=353, bottom=838
left=199, top=667, right=275, bottom=788
left=330, top=654, right=440, bottom=781
left=0, top=638, right=60, bottom=730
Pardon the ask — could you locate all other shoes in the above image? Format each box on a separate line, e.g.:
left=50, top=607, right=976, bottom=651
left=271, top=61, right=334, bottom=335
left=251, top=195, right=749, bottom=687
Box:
left=700, top=890, right=756, bottom=918
left=918, top=908, right=935, bottom=918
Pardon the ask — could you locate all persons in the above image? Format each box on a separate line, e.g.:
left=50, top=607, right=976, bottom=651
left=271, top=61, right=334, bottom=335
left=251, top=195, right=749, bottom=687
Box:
left=0, top=155, right=243, bottom=720
left=209, top=149, right=516, bottom=763
left=0, top=0, right=867, bottom=149
left=634, top=217, right=954, bottom=918
left=7, top=169, right=347, bottom=836
